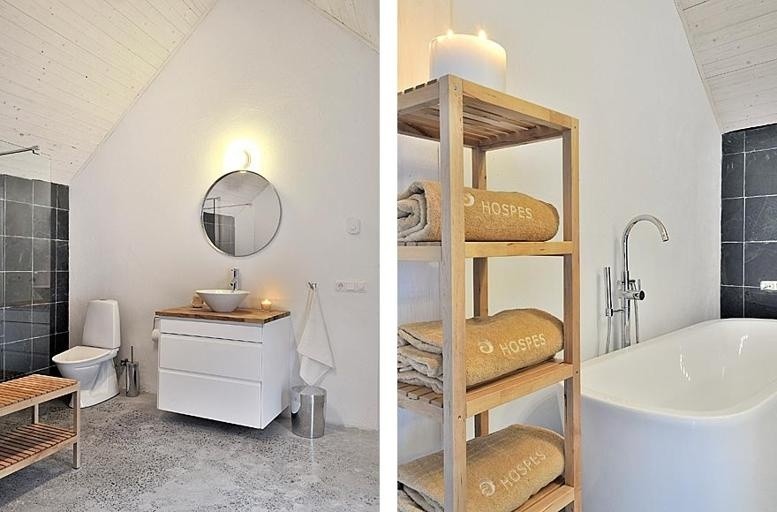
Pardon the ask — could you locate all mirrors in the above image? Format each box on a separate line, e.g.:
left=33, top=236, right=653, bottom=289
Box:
left=200, top=169, right=280, bottom=257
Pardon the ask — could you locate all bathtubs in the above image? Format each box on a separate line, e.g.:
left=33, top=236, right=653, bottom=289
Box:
left=556, top=317, right=777, bottom=511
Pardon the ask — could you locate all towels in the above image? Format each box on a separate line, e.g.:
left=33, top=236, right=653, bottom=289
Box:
left=398, top=309, right=564, bottom=394
left=398, top=424, right=565, bottom=512
left=297, top=287, right=333, bottom=386
left=397, top=180, right=559, bottom=242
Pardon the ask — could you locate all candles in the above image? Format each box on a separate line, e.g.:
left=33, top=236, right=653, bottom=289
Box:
left=428, top=29, right=506, bottom=94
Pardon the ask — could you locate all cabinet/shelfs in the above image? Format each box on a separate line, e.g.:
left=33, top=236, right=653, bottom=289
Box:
left=157, top=316, right=291, bottom=430
left=0, top=374, right=81, bottom=479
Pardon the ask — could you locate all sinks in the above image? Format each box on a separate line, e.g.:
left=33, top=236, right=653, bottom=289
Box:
left=195, top=289, right=251, bottom=313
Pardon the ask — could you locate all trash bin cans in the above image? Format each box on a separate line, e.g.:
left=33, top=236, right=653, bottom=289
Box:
left=290, top=386, right=327, bottom=439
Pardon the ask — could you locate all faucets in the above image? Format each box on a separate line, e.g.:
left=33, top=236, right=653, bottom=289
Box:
left=228, top=267, right=241, bottom=290
left=618, top=215, right=670, bottom=347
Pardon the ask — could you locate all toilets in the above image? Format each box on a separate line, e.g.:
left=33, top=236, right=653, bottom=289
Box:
left=50, top=298, right=121, bottom=410
